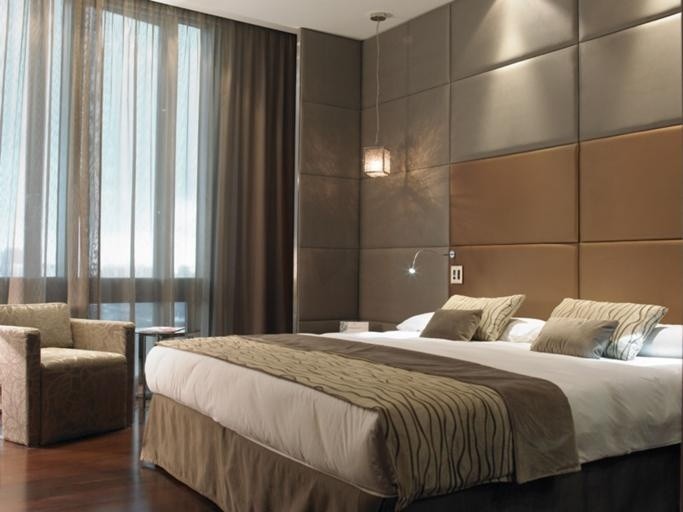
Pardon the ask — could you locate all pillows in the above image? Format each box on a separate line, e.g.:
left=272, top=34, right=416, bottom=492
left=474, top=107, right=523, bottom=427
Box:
left=420, top=308, right=483, bottom=341
left=522, top=323, right=682, bottom=359
left=441, top=294, right=526, bottom=341
left=530, top=318, right=620, bottom=359
left=548, top=298, right=670, bottom=360
left=396, top=311, right=547, bottom=344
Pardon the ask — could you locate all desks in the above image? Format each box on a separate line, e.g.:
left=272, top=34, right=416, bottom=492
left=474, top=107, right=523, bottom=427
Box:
left=135, top=326, right=201, bottom=400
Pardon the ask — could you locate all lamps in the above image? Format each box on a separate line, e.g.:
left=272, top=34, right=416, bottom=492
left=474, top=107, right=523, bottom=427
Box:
left=361, top=12, right=391, bottom=178
left=408, top=249, right=455, bottom=274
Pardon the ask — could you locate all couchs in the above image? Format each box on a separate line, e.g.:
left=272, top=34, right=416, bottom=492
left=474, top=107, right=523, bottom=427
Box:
left=1, top=302, right=135, bottom=447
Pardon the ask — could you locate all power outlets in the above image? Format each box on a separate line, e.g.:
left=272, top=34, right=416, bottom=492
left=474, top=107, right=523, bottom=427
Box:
left=450, top=264, right=464, bottom=285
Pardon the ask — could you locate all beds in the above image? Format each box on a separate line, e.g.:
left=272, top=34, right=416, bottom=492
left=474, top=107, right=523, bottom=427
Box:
left=140, top=331, right=683, bottom=512
